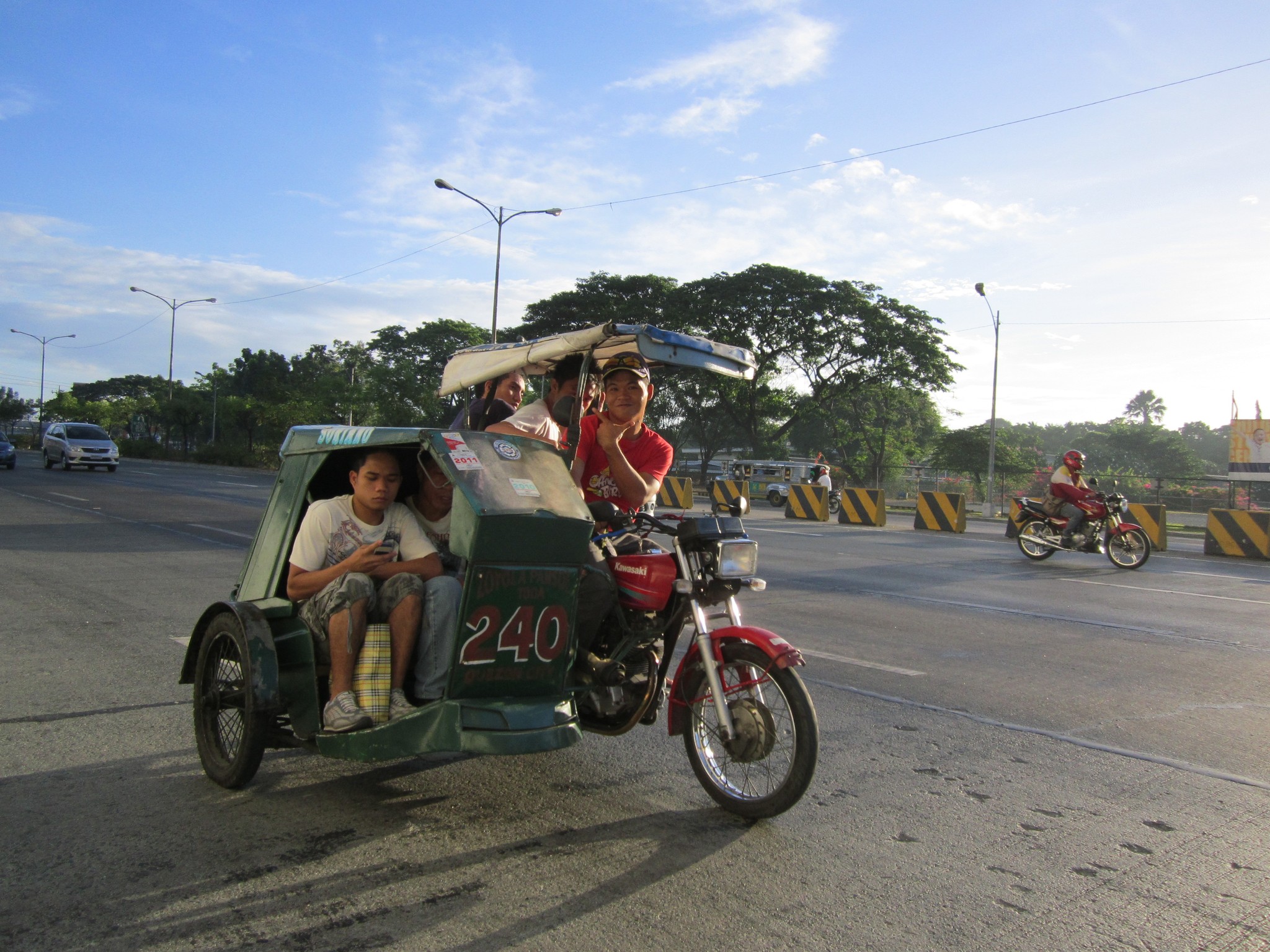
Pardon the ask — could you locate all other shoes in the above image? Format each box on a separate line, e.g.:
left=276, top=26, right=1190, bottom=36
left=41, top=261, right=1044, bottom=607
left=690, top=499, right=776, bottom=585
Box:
left=1060, top=537, right=1076, bottom=546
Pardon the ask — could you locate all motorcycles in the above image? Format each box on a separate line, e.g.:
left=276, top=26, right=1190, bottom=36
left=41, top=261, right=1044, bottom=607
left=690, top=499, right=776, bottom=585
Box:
left=1013, top=478, right=1152, bottom=569
left=829, top=489, right=843, bottom=515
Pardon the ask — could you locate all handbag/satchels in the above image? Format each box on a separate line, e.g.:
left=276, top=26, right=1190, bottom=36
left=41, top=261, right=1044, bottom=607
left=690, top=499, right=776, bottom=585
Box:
left=353, top=623, right=391, bottom=727
left=1041, top=492, right=1065, bottom=518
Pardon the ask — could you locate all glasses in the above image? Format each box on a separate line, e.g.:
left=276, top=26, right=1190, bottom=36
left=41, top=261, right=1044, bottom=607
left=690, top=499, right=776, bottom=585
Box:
left=603, top=357, right=650, bottom=382
left=436, top=540, right=456, bottom=572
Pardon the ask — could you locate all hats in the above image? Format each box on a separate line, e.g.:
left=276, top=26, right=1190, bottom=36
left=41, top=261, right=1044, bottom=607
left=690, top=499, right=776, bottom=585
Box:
left=601, top=351, right=650, bottom=383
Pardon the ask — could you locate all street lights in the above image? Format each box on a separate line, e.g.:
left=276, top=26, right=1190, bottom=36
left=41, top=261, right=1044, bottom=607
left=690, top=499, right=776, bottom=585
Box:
left=11, top=329, right=77, bottom=451
left=516, top=335, right=545, bottom=400
left=130, top=287, right=216, bottom=459
left=435, top=178, right=562, bottom=344
left=176, top=319, right=819, bottom=820
left=194, top=371, right=216, bottom=448
left=974, top=283, right=999, bottom=517
left=320, top=353, right=354, bottom=426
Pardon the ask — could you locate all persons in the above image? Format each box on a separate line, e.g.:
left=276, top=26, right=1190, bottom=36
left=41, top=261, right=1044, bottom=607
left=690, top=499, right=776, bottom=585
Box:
left=812, top=467, right=832, bottom=491
left=1245, top=428, right=1270, bottom=463
left=401, top=454, right=462, bottom=700
left=450, top=371, right=525, bottom=430
left=286, top=446, right=443, bottom=731
left=559, top=351, right=673, bottom=554
left=485, top=353, right=603, bottom=450
left=1049, top=450, right=1097, bottom=546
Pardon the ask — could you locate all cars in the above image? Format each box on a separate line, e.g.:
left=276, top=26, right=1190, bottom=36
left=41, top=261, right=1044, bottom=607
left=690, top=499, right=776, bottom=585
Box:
left=0, top=430, right=16, bottom=469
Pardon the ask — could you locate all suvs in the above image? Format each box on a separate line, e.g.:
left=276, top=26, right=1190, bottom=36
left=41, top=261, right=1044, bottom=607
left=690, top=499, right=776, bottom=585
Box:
left=42, top=423, right=119, bottom=472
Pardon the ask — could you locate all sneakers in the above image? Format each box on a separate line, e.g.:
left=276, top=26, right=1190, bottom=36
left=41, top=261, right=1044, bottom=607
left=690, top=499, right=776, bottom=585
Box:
left=322, top=691, right=374, bottom=732
left=388, top=689, right=417, bottom=720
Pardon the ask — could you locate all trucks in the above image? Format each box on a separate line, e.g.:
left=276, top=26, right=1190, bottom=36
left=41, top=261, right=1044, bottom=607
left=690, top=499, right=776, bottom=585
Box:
left=715, top=460, right=830, bottom=507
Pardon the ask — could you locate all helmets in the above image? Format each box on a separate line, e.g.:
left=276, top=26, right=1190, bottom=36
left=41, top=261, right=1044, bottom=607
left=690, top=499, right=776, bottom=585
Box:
left=1063, top=450, right=1087, bottom=470
left=819, top=469, right=827, bottom=474
